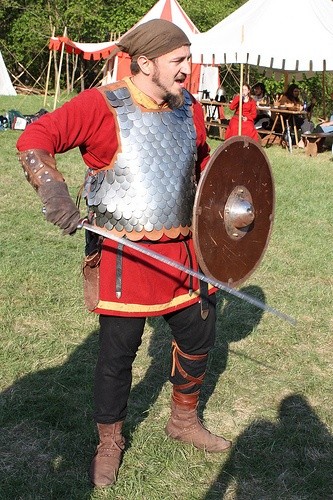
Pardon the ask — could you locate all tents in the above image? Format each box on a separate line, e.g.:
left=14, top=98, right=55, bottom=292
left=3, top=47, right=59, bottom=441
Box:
left=50, top=0, right=333, bottom=121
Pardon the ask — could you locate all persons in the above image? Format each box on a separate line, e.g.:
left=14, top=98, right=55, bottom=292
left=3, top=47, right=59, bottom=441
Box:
left=250, top=83, right=315, bottom=144
left=16, top=19, right=232, bottom=487
left=224, top=83, right=260, bottom=143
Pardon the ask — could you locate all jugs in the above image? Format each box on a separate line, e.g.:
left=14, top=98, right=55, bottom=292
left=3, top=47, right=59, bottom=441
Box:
left=301, top=119, right=313, bottom=134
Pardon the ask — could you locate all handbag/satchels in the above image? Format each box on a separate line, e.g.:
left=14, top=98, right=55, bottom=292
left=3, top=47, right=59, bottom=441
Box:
left=301, top=118, right=314, bottom=134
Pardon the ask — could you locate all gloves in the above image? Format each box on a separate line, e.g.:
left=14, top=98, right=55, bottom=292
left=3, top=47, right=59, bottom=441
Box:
left=37, top=180, right=81, bottom=234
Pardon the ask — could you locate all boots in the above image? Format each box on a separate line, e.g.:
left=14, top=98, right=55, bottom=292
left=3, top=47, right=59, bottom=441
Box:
left=90, top=421, right=126, bottom=486
left=165, top=401, right=232, bottom=452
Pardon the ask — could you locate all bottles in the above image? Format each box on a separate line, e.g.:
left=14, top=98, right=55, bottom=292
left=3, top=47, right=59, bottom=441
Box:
left=303, top=101, right=307, bottom=111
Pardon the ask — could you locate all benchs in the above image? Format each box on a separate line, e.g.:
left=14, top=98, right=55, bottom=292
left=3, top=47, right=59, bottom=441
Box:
left=301, top=134, right=333, bottom=159
left=204, top=121, right=275, bottom=141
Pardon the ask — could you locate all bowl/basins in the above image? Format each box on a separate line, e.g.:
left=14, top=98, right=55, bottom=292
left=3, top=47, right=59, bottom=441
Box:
left=280, top=106, right=287, bottom=110
left=288, top=107, right=294, bottom=110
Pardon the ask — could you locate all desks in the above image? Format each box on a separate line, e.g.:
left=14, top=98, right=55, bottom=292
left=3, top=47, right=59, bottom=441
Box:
left=199, top=99, right=308, bottom=149
